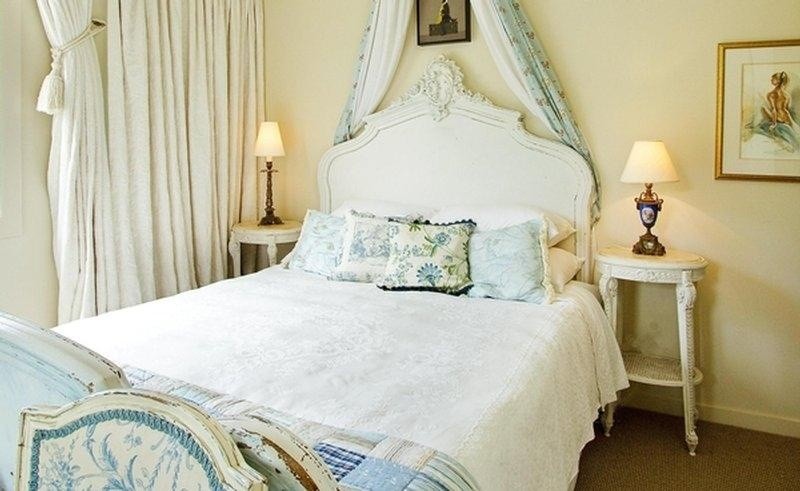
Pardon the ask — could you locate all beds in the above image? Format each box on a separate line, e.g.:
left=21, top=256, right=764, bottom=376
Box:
left=1, top=57, right=631, bottom=490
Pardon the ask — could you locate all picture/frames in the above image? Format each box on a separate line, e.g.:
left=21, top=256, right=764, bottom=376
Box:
left=416, top=0, right=470, bottom=46
left=714, top=39, right=800, bottom=182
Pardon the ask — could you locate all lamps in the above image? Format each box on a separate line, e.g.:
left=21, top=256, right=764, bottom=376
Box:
left=254, top=121, right=286, bottom=225
left=620, top=140, right=679, bottom=256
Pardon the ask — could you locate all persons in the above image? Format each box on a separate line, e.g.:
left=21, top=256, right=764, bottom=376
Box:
left=760, top=72, right=792, bottom=130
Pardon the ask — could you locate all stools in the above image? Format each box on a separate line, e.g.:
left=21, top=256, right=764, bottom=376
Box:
left=593, top=247, right=709, bottom=456
left=228, top=221, right=302, bottom=278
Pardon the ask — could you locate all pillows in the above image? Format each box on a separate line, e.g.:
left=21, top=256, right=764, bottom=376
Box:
left=284, top=208, right=583, bottom=305
